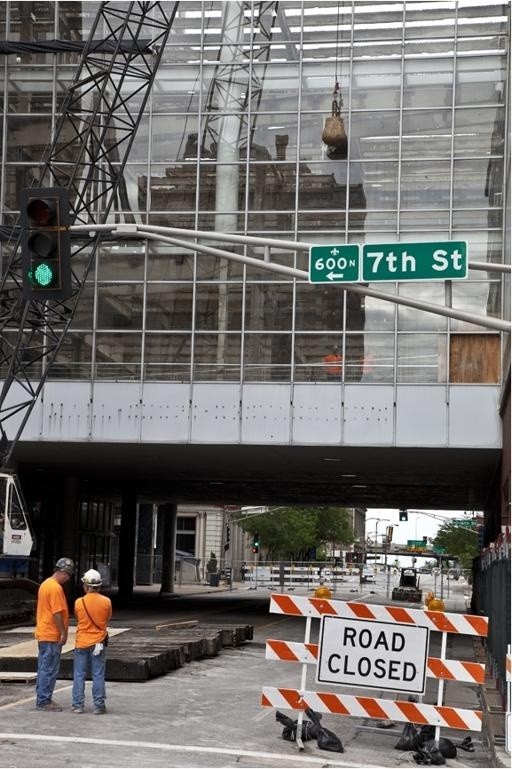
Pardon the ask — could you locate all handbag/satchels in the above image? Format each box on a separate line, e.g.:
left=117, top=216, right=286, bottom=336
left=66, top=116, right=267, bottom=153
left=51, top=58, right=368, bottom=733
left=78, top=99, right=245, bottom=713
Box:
left=104, top=633, right=109, bottom=647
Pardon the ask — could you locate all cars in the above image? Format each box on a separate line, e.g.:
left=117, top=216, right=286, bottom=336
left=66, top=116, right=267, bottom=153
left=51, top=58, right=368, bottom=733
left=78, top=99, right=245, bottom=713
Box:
left=359, top=567, right=376, bottom=584
left=431, top=566, right=461, bottom=582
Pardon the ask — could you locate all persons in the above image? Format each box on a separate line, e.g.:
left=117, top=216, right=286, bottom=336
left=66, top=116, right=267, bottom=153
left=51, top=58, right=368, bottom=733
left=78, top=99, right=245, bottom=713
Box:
left=323, top=344, right=345, bottom=382
left=239, top=562, right=249, bottom=583
left=70, top=568, right=115, bottom=714
left=359, top=347, right=376, bottom=384
left=31, top=557, right=79, bottom=710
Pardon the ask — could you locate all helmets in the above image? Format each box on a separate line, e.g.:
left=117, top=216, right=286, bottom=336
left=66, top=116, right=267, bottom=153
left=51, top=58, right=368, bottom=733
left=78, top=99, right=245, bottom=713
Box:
left=81, top=569, right=103, bottom=587
left=56, top=558, right=76, bottom=576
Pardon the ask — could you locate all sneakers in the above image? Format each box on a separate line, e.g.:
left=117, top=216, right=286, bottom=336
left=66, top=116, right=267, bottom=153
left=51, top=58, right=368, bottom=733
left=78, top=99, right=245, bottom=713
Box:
left=71, top=707, right=83, bottom=713
left=36, top=700, right=62, bottom=712
left=93, top=709, right=106, bottom=714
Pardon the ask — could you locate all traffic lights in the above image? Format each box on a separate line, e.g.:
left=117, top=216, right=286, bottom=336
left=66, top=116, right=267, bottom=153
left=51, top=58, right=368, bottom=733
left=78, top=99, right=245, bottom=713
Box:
left=398, top=510, right=409, bottom=523
left=251, top=532, right=260, bottom=556
left=22, top=188, right=73, bottom=299
left=226, top=525, right=231, bottom=544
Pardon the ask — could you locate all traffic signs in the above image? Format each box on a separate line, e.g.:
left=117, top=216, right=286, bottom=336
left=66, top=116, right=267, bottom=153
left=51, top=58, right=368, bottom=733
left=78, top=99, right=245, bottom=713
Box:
left=453, top=520, right=477, bottom=527
left=315, top=613, right=430, bottom=695
left=310, top=243, right=362, bottom=284
left=362, top=241, right=466, bottom=282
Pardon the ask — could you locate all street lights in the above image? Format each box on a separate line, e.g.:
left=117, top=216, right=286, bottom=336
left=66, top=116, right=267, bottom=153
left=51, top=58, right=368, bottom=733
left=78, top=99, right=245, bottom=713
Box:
left=365, top=516, right=399, bottom=571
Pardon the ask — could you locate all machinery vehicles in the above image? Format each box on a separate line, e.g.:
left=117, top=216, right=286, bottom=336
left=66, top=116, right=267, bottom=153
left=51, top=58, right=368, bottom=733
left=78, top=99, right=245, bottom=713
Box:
left=1, top=2, right=184, bottom=628
left=392, top=565, right=424, bottom=601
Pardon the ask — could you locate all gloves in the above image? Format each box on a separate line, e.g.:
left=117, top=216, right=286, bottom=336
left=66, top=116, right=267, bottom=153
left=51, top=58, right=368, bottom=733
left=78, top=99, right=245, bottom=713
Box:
left=93, top=643, right=103, bottom=656
